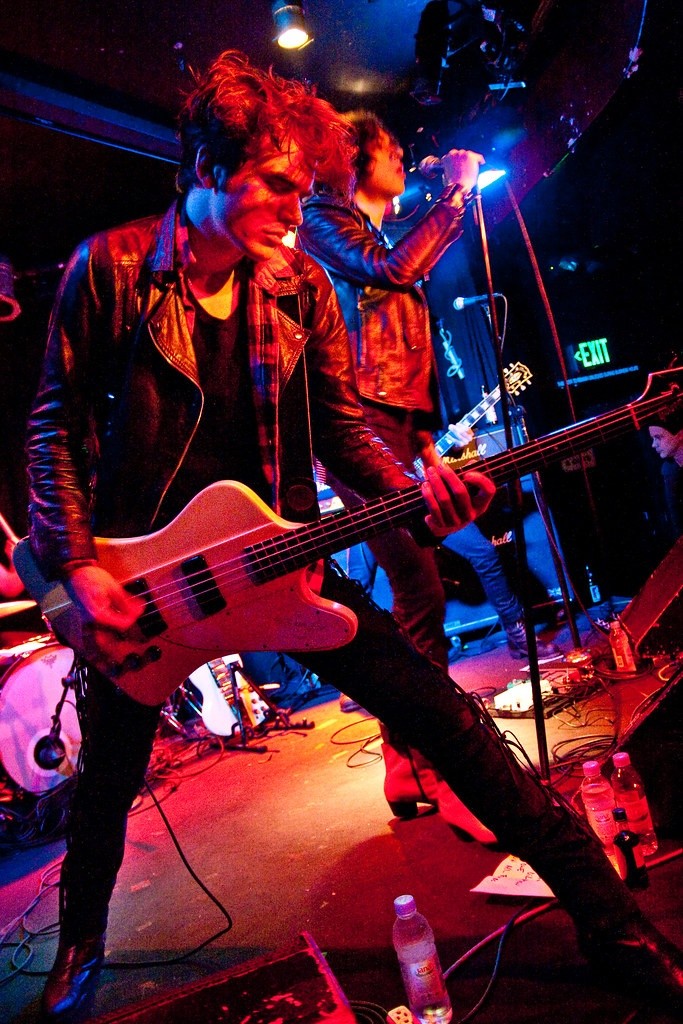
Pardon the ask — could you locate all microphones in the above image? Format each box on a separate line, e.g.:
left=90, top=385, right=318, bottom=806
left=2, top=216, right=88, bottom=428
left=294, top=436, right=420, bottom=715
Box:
left=419, top=155, right=447, bottom=179
left=452, top=293, right=499, bottom=311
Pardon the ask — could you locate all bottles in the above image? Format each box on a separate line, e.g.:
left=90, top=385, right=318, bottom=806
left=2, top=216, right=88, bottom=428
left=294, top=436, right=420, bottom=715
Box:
left=581, top=761, right=617, bottom=857
left=612, top=808, right=649, bottom=891
left=611, top=752, right=658, bottom=856
left=610, top=622, right=637, bottom=673
left=392, top=895, right=451, bottom=1024
left=585, top=565, right=603, bottom=605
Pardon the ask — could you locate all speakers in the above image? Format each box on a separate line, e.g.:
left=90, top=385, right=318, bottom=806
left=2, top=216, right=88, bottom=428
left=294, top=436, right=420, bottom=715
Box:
left=78, top=930, right=357, bottom=1024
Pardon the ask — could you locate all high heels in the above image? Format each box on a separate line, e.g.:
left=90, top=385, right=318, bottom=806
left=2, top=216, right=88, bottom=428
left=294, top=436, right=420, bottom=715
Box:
left=381, top=739, right=500, bottom=849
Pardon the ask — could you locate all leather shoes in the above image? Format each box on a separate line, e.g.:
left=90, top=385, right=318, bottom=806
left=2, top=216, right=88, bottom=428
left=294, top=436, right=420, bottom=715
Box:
left=580, top=919, right=683, bottom=1024
left=506, top=618, right=560, bottom=659
left=42, top=938, right=105, bottom=1019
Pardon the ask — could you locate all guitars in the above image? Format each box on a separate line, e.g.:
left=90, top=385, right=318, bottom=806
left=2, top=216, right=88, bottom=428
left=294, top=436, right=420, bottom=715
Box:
left=314, top=360, right=534, bottom=515
left=12, top=359, right=683, bottom=707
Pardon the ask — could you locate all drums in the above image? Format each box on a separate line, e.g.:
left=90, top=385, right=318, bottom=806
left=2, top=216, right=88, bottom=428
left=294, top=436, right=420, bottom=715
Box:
left=0, top=631, right=90, bottom=804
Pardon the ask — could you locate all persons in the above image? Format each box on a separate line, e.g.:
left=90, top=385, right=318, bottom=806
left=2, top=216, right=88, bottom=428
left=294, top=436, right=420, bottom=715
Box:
left=340, top=424, right=561, bottom=710
left=26, top=51, right=683, bottom=1023
left=648, top=408, right=683, bottom=538
left=299, top=108, right=560, bottom=852
